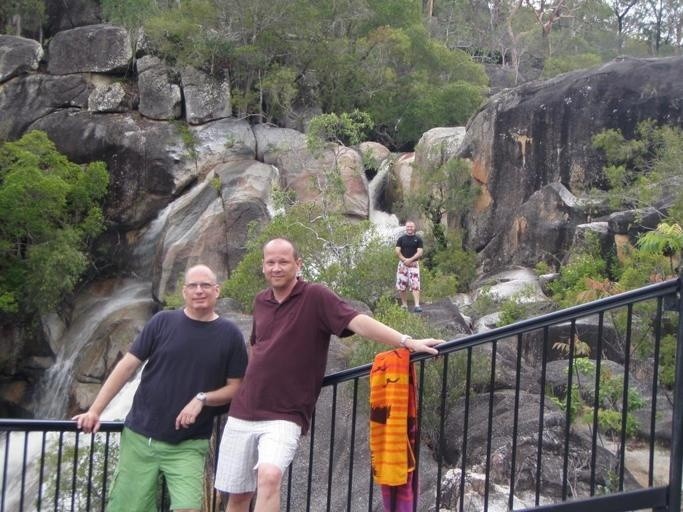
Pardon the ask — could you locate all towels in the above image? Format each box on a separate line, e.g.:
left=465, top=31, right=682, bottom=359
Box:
left=368, top=348, right=418, bottom=511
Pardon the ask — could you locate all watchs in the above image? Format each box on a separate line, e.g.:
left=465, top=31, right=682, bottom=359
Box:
left=400, top=334, right=412, bottom=348
left=195, top=392, right=208, bottom=406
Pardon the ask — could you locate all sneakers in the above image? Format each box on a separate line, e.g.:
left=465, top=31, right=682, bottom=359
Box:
left=415, top=307, right=422, bottom=312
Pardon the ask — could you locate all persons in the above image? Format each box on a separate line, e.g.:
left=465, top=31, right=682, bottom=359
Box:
left=396, top=220, right=423, bottom=312
left=214, top=238, right=446, bottom=512
left=70, top=264, right=248, bottom=512
